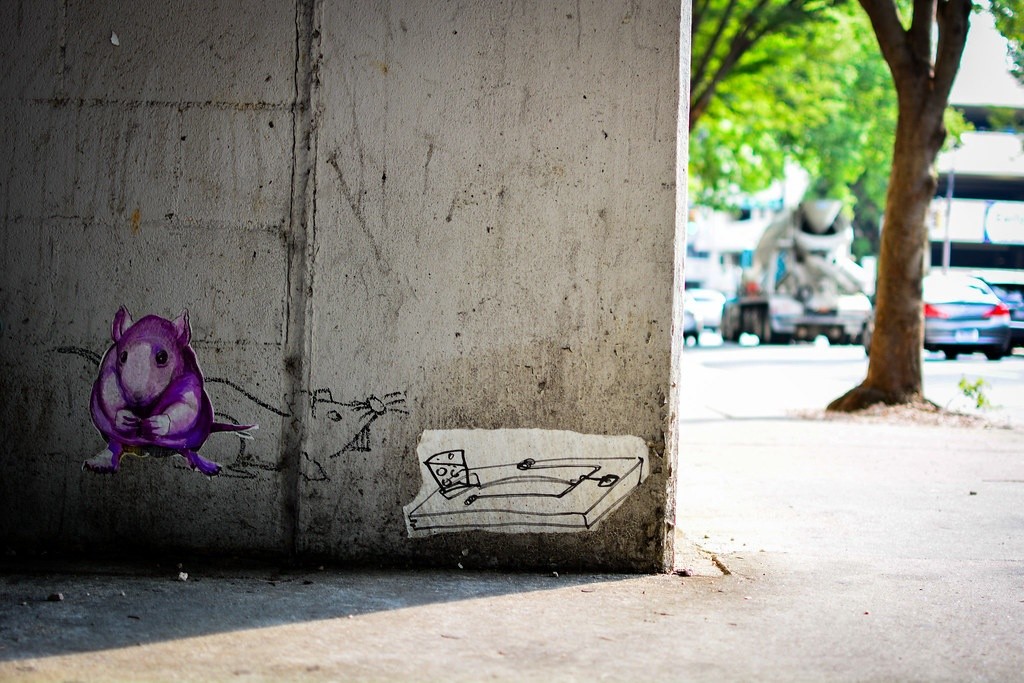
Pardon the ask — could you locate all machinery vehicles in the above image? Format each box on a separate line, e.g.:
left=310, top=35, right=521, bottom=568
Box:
left=716, top=174, right=874, bottom=346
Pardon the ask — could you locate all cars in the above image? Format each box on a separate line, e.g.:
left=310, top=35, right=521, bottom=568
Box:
left=862, top=270, right=1013, bottom=361
left=686, top=279, right=728, bottom=331
